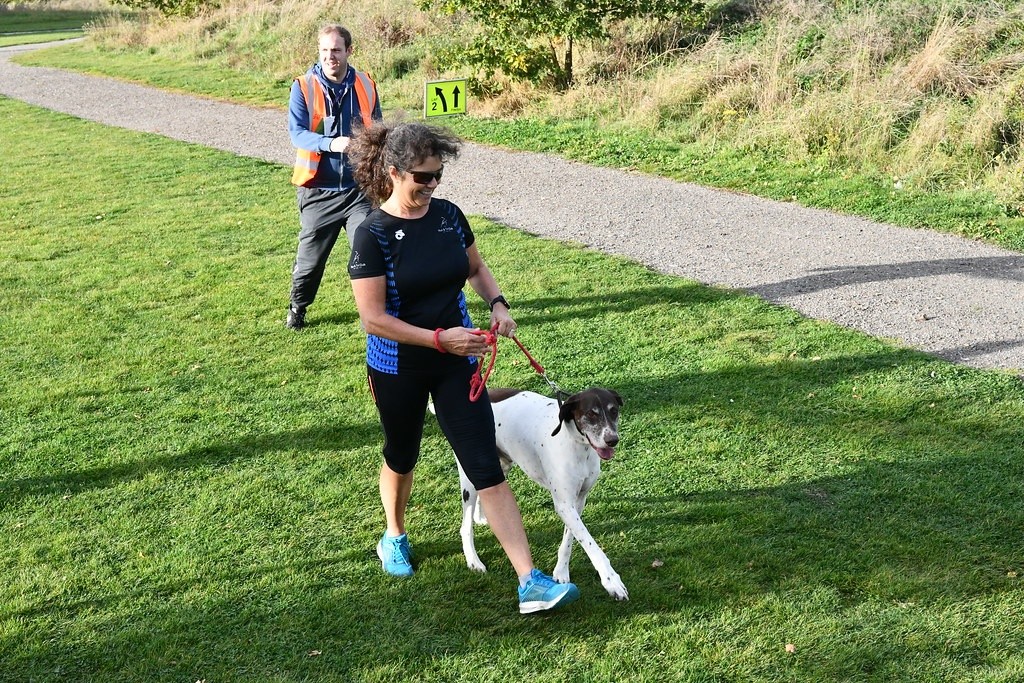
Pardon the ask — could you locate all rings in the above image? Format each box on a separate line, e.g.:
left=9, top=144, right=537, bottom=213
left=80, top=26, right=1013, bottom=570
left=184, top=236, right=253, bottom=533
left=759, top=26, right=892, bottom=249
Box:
left=510, top=329, right=516, bottom=332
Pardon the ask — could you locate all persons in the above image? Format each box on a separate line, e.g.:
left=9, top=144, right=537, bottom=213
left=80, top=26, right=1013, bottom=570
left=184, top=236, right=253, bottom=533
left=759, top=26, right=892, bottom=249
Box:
left=287, top=26, right=578, bottom=616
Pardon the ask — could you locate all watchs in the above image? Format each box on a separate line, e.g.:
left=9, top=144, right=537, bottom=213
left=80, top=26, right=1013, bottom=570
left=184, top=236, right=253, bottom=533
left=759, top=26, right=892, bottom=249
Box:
left=489, top=295, right=510, bottom=312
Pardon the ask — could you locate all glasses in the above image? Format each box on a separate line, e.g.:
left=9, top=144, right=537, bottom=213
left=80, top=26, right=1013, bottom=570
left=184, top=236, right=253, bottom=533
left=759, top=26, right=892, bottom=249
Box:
left=405, top=164, right=445, bottom=184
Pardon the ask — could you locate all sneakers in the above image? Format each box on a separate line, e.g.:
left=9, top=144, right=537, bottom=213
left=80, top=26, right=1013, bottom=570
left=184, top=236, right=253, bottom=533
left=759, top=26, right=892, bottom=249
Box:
left=377, top=530, right=416, bottom=579
left=517, top=569, right=581, bottom=613
left=286, top=304, right=307, bottom=332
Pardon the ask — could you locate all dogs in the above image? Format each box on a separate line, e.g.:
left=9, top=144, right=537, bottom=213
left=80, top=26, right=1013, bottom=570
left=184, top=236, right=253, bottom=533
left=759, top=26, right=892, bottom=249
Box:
left=429, top=388, right=629, bottom=602
